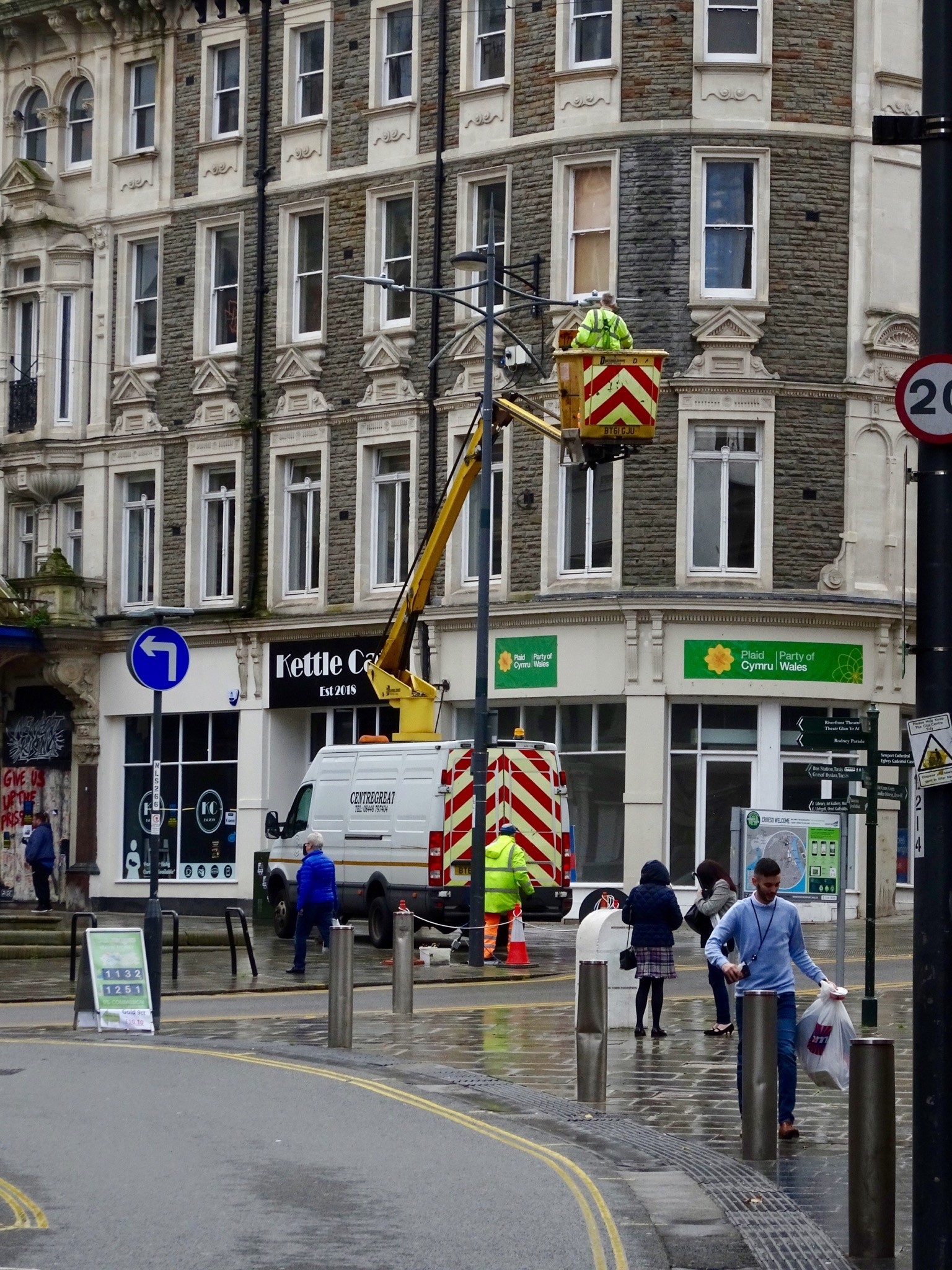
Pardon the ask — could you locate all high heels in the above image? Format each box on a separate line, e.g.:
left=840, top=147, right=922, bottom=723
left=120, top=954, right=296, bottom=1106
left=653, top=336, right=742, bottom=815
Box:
left=704, top=1023, right=734, bottom=1036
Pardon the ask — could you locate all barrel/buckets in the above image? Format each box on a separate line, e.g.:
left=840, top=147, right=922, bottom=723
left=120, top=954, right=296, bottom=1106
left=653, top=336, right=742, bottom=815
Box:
left=429, top=947, right=451, bottom=966
left=419, top=946, right=437, bottom=965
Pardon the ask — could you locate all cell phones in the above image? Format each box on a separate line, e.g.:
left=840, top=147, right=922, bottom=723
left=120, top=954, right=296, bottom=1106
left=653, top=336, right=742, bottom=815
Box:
left=725, top=965, right=751, bottom=984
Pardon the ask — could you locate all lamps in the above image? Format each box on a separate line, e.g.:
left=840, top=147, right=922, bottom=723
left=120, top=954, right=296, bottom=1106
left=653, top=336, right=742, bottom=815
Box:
left=448, top=247, right=540, bottom=319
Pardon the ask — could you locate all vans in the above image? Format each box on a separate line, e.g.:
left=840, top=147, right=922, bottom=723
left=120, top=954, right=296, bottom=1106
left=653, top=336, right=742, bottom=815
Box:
left=265, top=738, right=576, bottom=949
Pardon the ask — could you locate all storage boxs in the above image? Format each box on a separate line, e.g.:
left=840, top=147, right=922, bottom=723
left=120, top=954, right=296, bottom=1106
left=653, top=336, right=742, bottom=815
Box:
left=420, top=946, right=452, bottom=965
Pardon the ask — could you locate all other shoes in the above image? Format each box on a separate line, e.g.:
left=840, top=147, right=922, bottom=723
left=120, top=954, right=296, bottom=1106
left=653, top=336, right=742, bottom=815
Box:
left=484, top=957, right=499, bottom=965
left=31, top=904, right=53, bottom=912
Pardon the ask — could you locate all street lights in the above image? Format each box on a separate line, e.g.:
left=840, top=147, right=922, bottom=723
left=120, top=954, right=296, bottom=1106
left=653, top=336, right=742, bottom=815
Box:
left=332, top=273, right=640, bottom=965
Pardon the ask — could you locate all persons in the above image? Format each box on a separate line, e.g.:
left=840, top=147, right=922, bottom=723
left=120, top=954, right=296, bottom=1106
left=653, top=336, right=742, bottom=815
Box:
left=24, top=812, right=56, bottom=913
left=684, top=859, right=737, bottom=1037
left=571, top=292, right=633, bottom=350
left=484, top=824, right=535, bottom=962
left=704, top=857, right=839, bottom=1140
left=619, top=860, right=683, bottom=1038
left=286, top=833, right=338, bottom=974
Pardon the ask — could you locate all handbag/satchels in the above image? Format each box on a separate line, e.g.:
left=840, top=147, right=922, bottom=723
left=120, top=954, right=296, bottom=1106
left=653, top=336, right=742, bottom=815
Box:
left=795, top=980, right=858, bottom=1091
left=684, top=890, right=713, bottom=936
left=620, top=946, right=637, bottom=970
left=322, top=918, right=341, bottom=953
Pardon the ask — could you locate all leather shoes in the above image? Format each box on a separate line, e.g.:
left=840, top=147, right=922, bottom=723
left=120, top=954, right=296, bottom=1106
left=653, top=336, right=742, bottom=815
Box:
left=778, top=1122, right=799, bottom=1140
left=740, top=1130, right=742, bottom=1137
left=635, top=1028, right=646, bottom=1036
left=286, top=967, right=305, bottom=975
left=651, top=1028, right=668, bottom=1037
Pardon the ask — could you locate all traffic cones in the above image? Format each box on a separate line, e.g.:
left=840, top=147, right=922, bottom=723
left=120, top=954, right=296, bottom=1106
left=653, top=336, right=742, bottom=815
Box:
left=600, top=892, right=620, bottom=909
left=495, top=904, right=540, bottom=970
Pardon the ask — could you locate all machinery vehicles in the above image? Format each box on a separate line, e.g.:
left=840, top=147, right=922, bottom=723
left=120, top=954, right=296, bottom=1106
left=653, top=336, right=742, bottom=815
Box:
left=368, top=337, right=673, bottom=742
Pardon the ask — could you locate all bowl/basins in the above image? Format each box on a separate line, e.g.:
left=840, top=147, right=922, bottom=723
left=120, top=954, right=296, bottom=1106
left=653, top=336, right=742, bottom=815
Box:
left=830, top=986, right=849, bottom=1000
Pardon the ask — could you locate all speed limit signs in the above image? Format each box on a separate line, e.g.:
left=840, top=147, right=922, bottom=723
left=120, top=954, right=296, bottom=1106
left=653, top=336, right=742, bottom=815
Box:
left=894, top=353, right=952, bottom=444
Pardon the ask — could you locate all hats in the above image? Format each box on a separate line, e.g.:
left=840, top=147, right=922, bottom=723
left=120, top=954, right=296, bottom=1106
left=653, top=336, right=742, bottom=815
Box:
left=500, top=824, right=521, bottom=833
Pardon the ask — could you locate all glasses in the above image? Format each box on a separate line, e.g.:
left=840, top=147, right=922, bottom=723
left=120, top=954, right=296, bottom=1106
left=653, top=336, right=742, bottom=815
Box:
left=305, top=843, right=310, bottom=846
